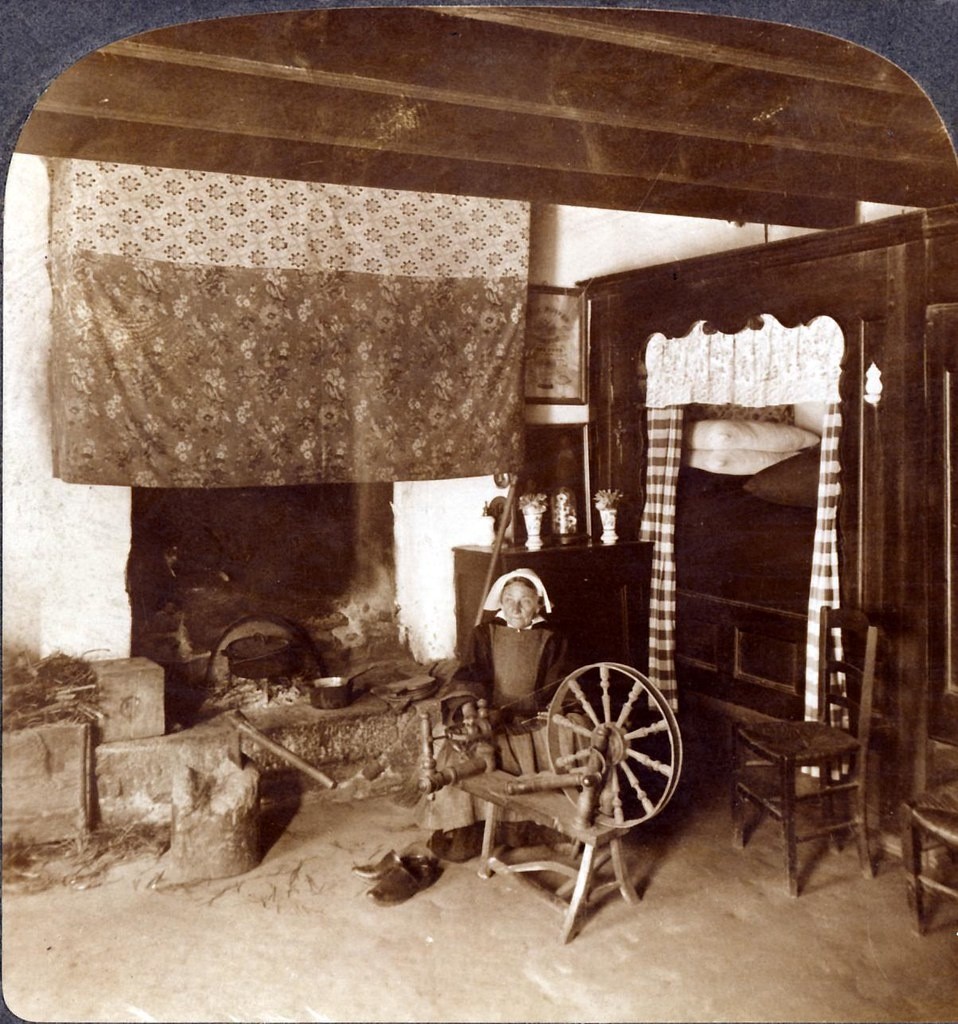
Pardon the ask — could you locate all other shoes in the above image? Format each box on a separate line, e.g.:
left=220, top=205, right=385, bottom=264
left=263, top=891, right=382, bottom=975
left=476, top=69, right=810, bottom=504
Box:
left=366, top=865, right=435, bottom=904
left=352, top=850, right=434, bottom=883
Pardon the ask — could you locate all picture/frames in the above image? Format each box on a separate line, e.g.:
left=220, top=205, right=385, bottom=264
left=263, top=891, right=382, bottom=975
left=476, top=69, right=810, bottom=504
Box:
left=522, top=282, right=591, bottom=406
left=513, top=423, right=587, bottom=544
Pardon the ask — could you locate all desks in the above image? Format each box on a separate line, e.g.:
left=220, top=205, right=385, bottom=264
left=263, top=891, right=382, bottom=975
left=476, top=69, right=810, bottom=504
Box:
left=452, top=540, right=650, bottom=709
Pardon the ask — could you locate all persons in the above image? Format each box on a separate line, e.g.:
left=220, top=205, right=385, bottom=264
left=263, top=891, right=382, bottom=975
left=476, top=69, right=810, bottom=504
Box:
left=426, top=567, right=575, bottom=863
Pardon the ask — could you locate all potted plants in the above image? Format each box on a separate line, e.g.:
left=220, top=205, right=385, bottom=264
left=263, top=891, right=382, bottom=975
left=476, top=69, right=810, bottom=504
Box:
left=517, top=493, right=548, bottom=536
left=592, top=488, right=624, bottom=530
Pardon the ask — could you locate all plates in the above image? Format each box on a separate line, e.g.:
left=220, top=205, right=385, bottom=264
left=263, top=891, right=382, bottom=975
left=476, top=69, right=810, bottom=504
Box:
left=376, top=673, right=440, bottom=706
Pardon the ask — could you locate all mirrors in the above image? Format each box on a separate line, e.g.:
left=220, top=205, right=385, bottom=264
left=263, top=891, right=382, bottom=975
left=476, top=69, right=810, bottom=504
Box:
left=0, top=8, right=957, bottom=1023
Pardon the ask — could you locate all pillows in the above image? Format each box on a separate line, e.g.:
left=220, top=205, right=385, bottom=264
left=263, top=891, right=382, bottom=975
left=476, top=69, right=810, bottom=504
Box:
left=682, top=419, right=821, bottom=453
left=680, top=449, right=802, bottom=476
left=743, top=449, right=818, bottom=509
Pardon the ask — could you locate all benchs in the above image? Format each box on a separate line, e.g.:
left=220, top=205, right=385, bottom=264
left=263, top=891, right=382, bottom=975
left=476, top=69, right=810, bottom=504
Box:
left=452, top=771, right=642, bottom=945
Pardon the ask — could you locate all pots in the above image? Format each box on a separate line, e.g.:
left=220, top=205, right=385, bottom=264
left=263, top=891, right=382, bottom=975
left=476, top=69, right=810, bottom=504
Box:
left=306, top=663, right=381, bottom=710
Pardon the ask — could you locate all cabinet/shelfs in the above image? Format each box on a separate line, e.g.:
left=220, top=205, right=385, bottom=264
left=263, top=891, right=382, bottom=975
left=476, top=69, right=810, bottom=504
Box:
left=582, top=212, right=925, bottom=736
left=857, top=205, right=958, bottom=868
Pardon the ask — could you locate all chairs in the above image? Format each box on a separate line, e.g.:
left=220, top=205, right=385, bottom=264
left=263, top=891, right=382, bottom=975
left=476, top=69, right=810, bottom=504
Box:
left=900, top=781, right=957, bottom=937
left=728, top=605, right=874, bottom=899
